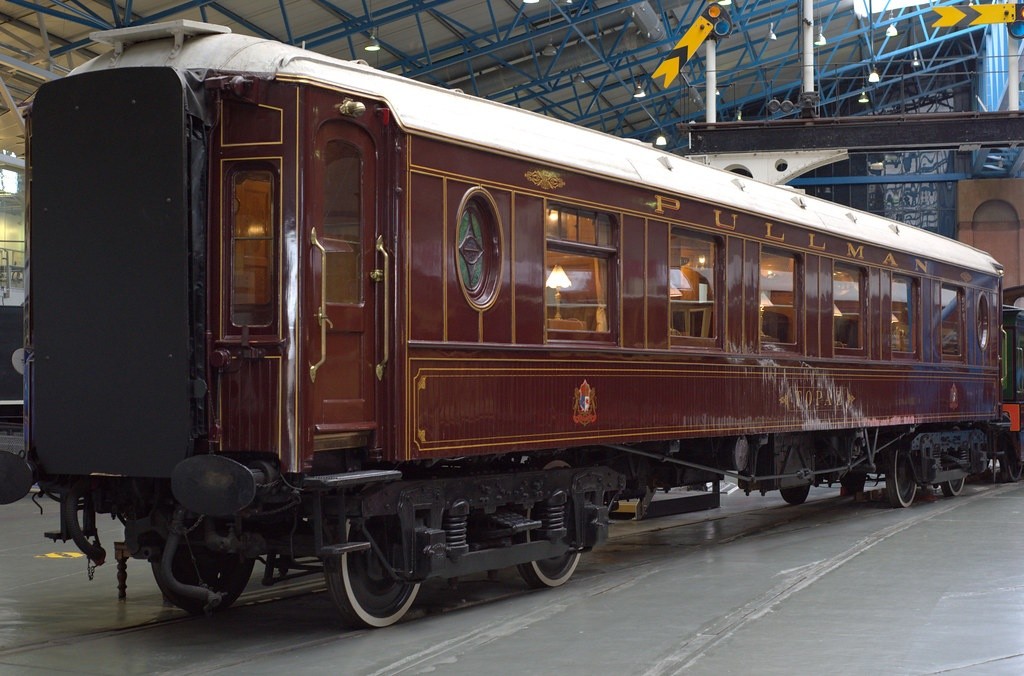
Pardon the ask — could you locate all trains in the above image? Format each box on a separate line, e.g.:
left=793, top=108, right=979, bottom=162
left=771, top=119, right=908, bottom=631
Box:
left=22, top=20, right=1024, bottom=629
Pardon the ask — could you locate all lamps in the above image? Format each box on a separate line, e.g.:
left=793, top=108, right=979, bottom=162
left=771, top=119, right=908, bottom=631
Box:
left=814, top=0, right=827, bottom=46
left=759, top=292, right=773, bottom=337
left=670, top=281, right=683, bottom=336
left=886, top=9, right=897, bottom=37
left=868, top=63, right=880, bottom=83
left=910, top=6, right=921, bottom=66
left=574, top=72, right=584, bottom=84
left=633, top=72, right=646, bottom=97
left=546, top=264, right=572, bottom=321
left=364, top=26, right=381, bottom=52
left=858, top=66, right=870, bottom=103
left=833, top=303, right=843, bottom=347
left=891, top=313, right=900, bottom=351
left=765, top=0, right=777, bottom=40
left=541, top=33, right=557, bottom=56
